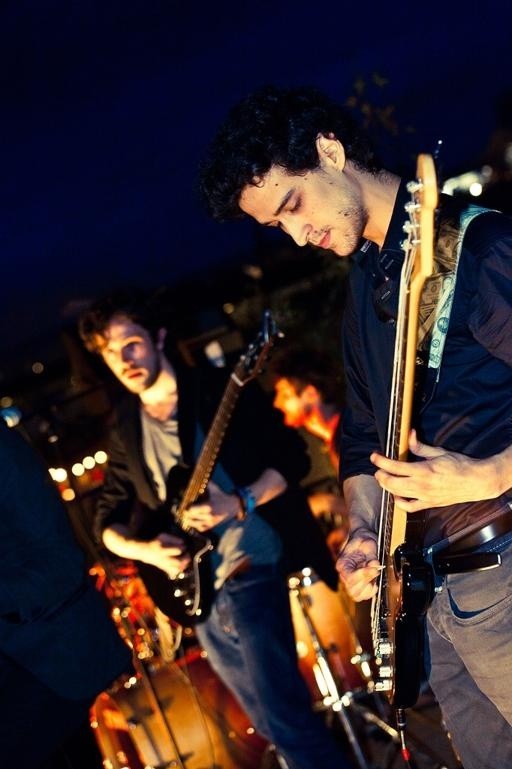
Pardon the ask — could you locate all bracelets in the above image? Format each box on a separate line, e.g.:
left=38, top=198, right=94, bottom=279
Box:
left=237, top=487, right=256, bottom=515
left=346, top=524, right=378, bottom=544
left=230, top=487, right=248, bottom=523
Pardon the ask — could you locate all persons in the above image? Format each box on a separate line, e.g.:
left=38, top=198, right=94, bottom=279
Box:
left=0, top=412, right=135, bottom=768
left=264, top=350, right=347, bottom=564
left=78, top=288, right=365, bottom=769
left=203, top=82, right=512, bottom=769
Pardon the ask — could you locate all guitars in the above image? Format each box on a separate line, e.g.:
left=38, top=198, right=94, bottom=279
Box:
left=134, top=312, right=282, bottom=626
left=358, top=153, right=437, bottom=704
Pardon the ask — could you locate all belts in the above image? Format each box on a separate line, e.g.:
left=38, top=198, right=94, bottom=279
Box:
left=433, top=513, right=511, bottom=575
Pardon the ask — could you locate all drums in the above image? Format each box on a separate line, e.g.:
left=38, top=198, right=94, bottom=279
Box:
left=287, top=575, right=367, bottom=706
left=90, top=661, right=283, bottom=765
